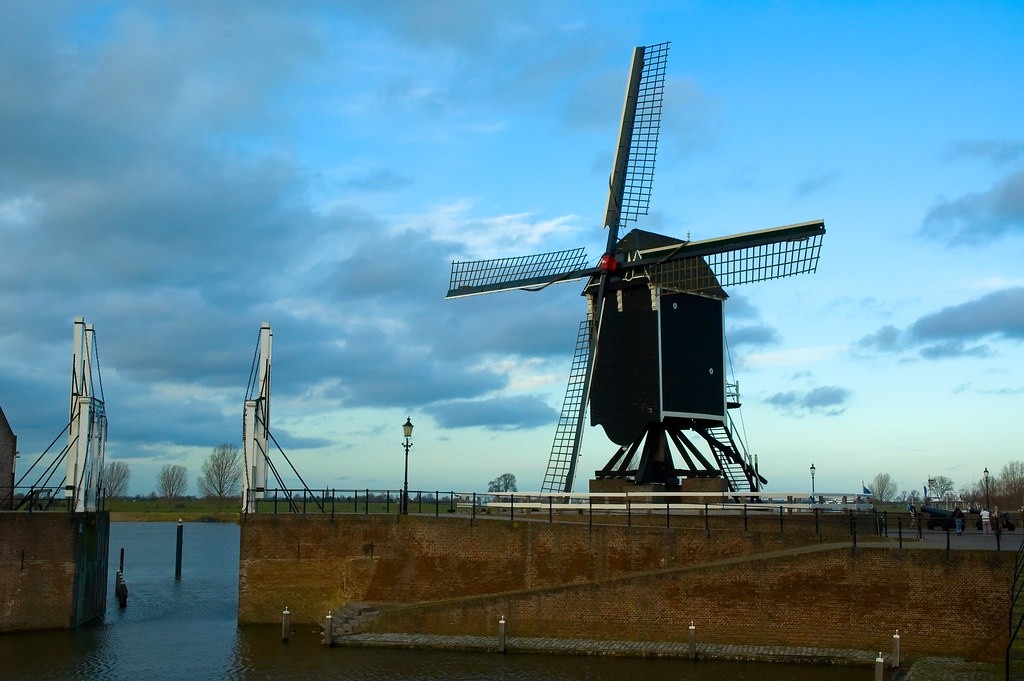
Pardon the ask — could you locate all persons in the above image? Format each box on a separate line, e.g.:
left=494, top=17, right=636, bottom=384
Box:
left=951, top=508, right=965, bottom=535
left=910, top=504, right=917, bottom=529
left=989, top=504, right=1006, bottom=550
left=980, top=508, right=994, bottom=537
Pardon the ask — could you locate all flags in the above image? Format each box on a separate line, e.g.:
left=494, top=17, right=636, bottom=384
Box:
left=864, top=486, right=870, bottom=493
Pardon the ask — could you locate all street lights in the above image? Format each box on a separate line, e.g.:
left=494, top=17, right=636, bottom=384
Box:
left=401, top=416, right=414, bottom=514
left=983, top=467, right=990, bottom=510
left=810, top=464, right=817, bottom=501
